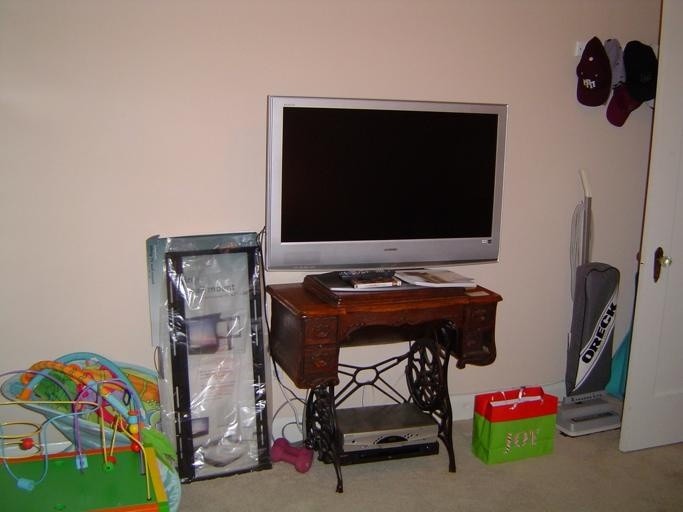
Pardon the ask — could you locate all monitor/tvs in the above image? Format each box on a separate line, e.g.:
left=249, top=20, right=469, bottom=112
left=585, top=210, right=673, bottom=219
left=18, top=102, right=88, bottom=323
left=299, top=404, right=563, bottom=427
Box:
left=263, top=94, right=509, bottom=293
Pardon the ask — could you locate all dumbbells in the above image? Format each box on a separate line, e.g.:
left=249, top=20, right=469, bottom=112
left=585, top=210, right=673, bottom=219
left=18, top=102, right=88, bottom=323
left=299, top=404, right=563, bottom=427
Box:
left=271, top=438, right=313, bottom=473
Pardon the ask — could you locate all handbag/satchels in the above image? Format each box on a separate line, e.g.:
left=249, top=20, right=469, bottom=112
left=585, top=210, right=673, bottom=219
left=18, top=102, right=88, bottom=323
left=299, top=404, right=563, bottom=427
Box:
left=472, top=387, right=558, bottom=466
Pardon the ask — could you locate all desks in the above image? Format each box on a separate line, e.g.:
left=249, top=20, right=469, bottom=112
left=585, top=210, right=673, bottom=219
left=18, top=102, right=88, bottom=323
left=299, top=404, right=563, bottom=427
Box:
left=266, top=282, right=503, bottom=494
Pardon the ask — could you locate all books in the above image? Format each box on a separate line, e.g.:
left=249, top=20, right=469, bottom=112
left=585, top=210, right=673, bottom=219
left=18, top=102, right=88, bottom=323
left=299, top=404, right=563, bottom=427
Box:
left=350, top=272, right=403, bottom=290
left=392, top=270, right=478, bottom=288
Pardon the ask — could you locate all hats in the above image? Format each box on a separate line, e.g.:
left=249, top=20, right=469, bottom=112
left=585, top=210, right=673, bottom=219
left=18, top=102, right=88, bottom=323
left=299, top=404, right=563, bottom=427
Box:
left=576, top=36, right=656, bottom=126
